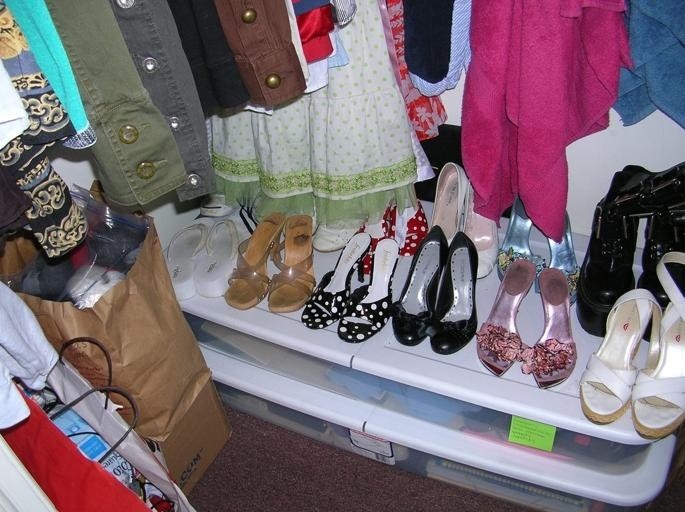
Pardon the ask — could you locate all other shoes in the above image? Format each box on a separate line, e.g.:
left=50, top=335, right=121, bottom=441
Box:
left=311, top=216, right=360, bottom=252
left=359, top=199, right=429, bottom=275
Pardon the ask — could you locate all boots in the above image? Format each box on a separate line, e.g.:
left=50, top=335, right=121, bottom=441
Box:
left=576, top=164, right=685, bottom=343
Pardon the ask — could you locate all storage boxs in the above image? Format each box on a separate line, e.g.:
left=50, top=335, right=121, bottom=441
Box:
left=182, top=314, right=678, bottom=512
left=162, top=200, right=675, bottom=478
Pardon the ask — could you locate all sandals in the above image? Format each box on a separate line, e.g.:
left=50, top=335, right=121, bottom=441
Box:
left=579, top=288, right=663, bottom=426
left=631, top=252, right=685, bottom=440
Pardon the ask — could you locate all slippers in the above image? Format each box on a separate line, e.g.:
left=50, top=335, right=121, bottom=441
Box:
left=194, top=216, right=245, bottom=298
left=167, top=217, right=214, bottom=301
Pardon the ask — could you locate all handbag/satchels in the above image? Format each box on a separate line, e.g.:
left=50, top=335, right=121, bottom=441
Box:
left=0, top=336, right=197, bottom=512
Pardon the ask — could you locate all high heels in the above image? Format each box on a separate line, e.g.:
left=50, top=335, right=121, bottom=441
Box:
left=267, top=215, right=316, bottom=314
left=338, top=236, right=400, bottom=343
left=496, top=196, right=534, bottom=283
left=521, top=268, right=577, bottom=390
left=392, top=224, right=449, bottom=347
left=301, top=233, right=373, bottom=330
left=225, top=212, right=287, bottom=310
left=535, top=210, right=581, bottom=308
left=425, top=231, right=479, bottom=355
left=428, top=163, right=469, bottom=249
left=462, top=179, right=499, bottom=279
left=477, top=260, right=537, bottom=378
